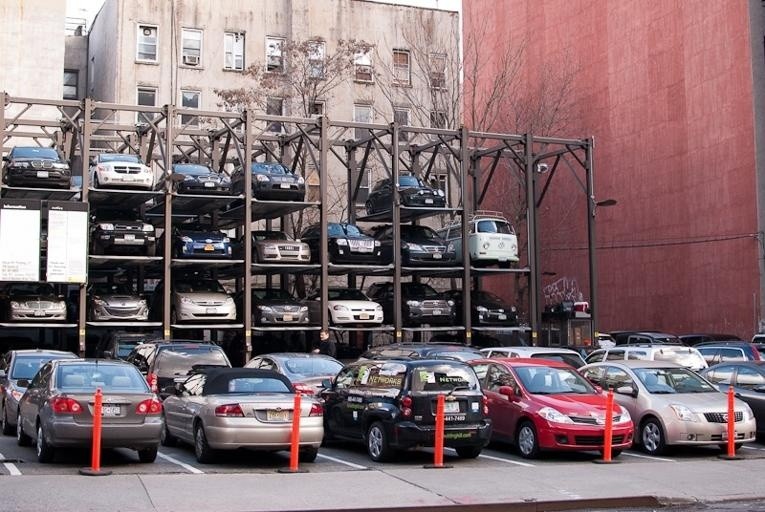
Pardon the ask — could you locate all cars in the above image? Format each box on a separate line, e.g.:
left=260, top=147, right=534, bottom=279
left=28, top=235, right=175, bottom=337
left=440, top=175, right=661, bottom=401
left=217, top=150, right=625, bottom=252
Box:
left=0, top=349, right=90, bottom=436
left=69, top=210, right=457, bottom=360
left=155, top=162, right=231, bottom=195
left=0, top=146, right=72, bottom=200
left=557, top=330, right=765, bottom=378
left=160, top=369, right=324, bottom=463
left=89, top=154, right=154, bottom=191
left=698, top=355, right=761, bottom=434
left=471, top=351, right=635, bottom=463
left=241, top=352, right=346, bottom=392
left=440, top=288, right=520, bottom=328
left=0, top=282, right=67, bottom=323
left=575, top=357, right=753, bottom=453
left=16, top=351, right=163, bottom=465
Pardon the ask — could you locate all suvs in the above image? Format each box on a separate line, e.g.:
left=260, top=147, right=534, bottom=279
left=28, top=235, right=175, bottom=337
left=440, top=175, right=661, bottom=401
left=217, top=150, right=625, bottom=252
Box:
left=125, top=339, right=232, bottom=400
left=426, top=331, right=529, bottom=356
left=356, top=342, right=485, bottom=362
left=323, top=356, right=497, bottom=456
left=365, top=174, right=446, bottom=215
left=480, top=347, right=588, bottom=387
left=229, top=162, right=305, bottom=203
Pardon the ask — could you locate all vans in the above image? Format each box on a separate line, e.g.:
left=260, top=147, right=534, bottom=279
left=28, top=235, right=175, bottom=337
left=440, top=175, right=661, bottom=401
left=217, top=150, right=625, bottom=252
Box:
left=430, top=210, right=520, bottom=269
